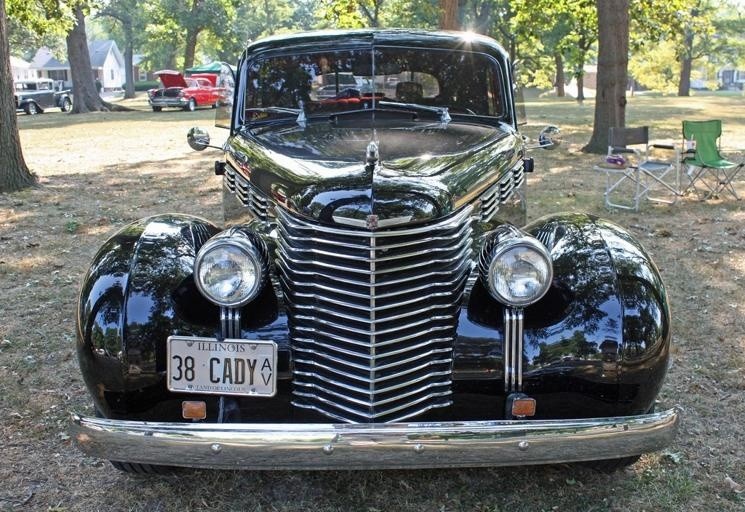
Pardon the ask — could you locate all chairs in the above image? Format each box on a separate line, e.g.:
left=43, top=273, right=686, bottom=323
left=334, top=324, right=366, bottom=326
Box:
left=595, top=125, right=682, bottom=212
left=676, top=119, right=744, bottom=204
left=395, top=79, right=424, bottom=104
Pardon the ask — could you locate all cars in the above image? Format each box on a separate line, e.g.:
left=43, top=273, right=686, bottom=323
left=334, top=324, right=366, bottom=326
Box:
left=11, top=78, right=71, bottom=115
left=309, top=71, right=406, bottom=103
left=68, top=29, right=683, bottom=469
left=145, top=66, right=229, bottom=112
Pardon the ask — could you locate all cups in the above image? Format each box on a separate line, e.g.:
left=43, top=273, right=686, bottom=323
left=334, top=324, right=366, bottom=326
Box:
left=686, top=140, right=696, bottom=159
